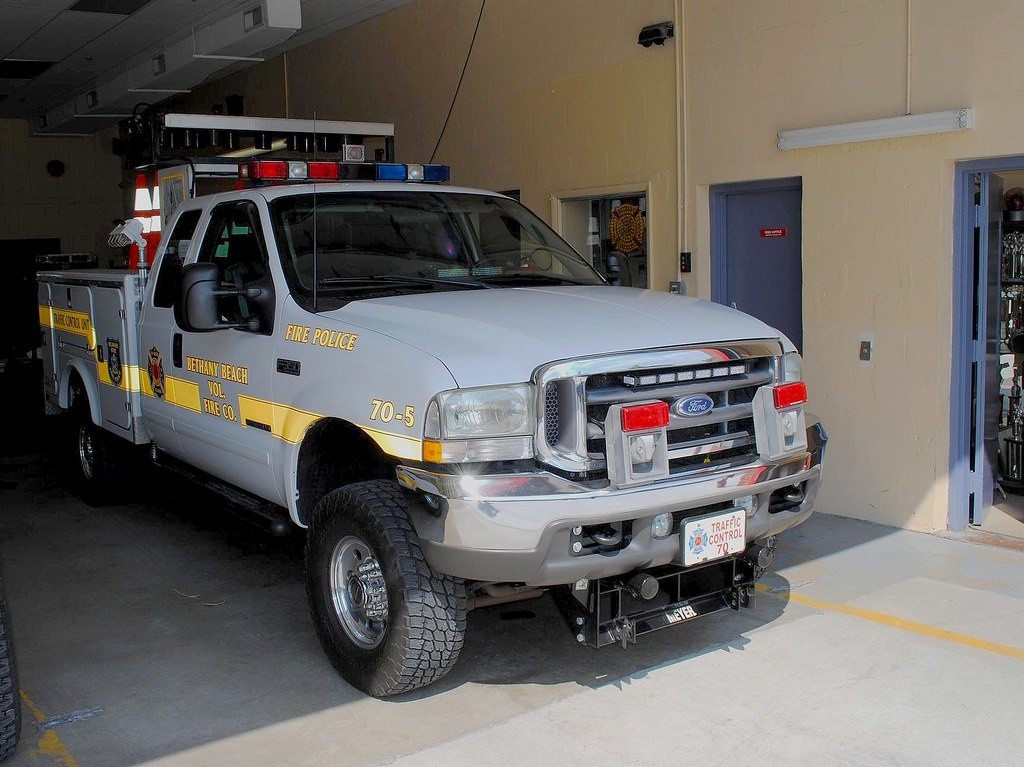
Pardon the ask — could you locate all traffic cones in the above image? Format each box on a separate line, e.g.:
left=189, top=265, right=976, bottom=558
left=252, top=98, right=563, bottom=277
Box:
left=127, top=173, right=161, bottom=271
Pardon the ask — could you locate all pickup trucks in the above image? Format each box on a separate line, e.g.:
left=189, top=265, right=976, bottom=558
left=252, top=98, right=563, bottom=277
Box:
left=36, top=102, right=828, bottom=700
